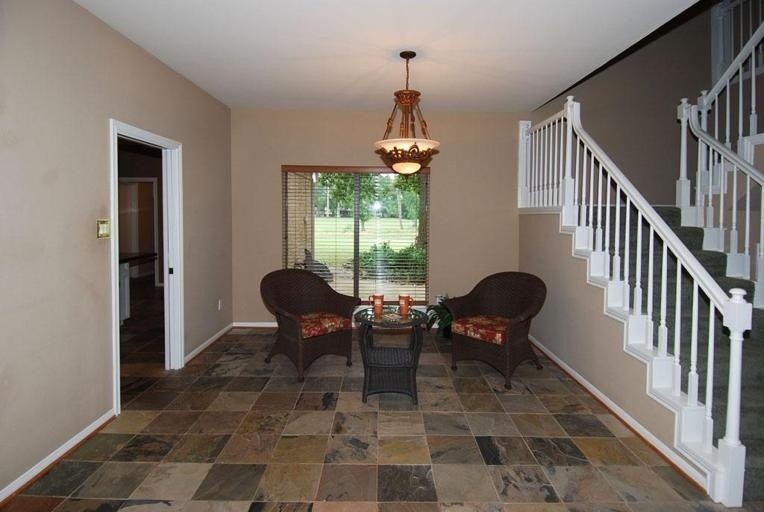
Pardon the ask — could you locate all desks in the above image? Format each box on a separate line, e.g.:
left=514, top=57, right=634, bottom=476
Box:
left=354, top=308, right=428, bottom=404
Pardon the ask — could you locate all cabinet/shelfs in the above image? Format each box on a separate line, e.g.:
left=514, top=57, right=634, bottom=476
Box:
left=119, top=261, right=130, bottom=325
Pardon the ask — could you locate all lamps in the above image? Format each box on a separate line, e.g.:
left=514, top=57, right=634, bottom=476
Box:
left=373, top=52, right=441, bottom=175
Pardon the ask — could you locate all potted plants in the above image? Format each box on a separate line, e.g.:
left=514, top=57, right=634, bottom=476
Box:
left=426, top=296, right=453, bottom=337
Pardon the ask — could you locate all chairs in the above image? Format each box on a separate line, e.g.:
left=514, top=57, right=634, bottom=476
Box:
left=444, top=272, right=547, bottom=389
left=261, top=268, right=361, bottom=382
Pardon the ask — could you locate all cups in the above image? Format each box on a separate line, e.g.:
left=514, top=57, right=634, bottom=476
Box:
left=369, top=294, right=384, bottom=313
left=399, top=294, right=413, bottom=315
